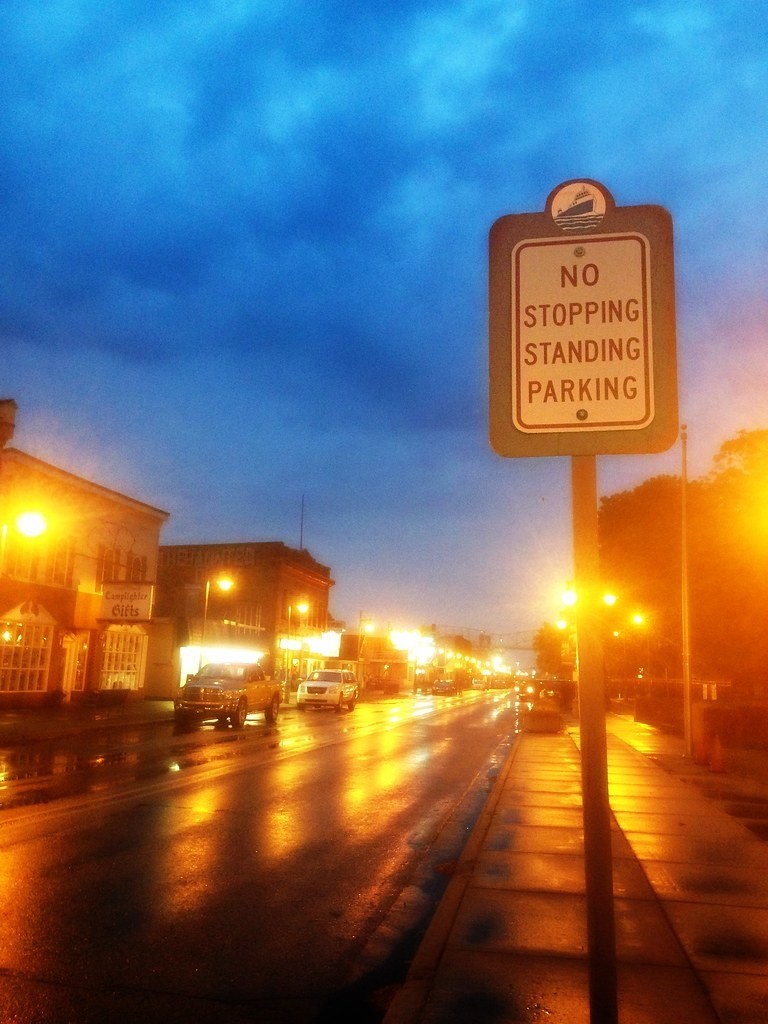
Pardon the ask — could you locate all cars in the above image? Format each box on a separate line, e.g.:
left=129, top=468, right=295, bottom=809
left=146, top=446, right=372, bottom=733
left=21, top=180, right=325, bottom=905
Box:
left=432, top=680, right=456, bottom=696
left=518, top=680, right=577, bottom=704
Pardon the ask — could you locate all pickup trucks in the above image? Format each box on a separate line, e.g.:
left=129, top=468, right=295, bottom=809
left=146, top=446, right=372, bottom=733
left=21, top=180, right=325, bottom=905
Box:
left=174, top=662, right=284, bottom=729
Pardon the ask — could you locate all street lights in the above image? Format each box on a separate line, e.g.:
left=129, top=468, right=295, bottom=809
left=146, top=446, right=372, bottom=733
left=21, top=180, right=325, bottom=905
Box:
left=286, top=603, right=310, bottom=703
left=0, top=513, right=45, bottom=567
left=199, top=577, right=234, bottom=670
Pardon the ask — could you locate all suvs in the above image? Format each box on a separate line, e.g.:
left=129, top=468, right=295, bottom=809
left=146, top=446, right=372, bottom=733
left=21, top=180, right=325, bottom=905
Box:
left=296, top=669, right=359, bottom=712
